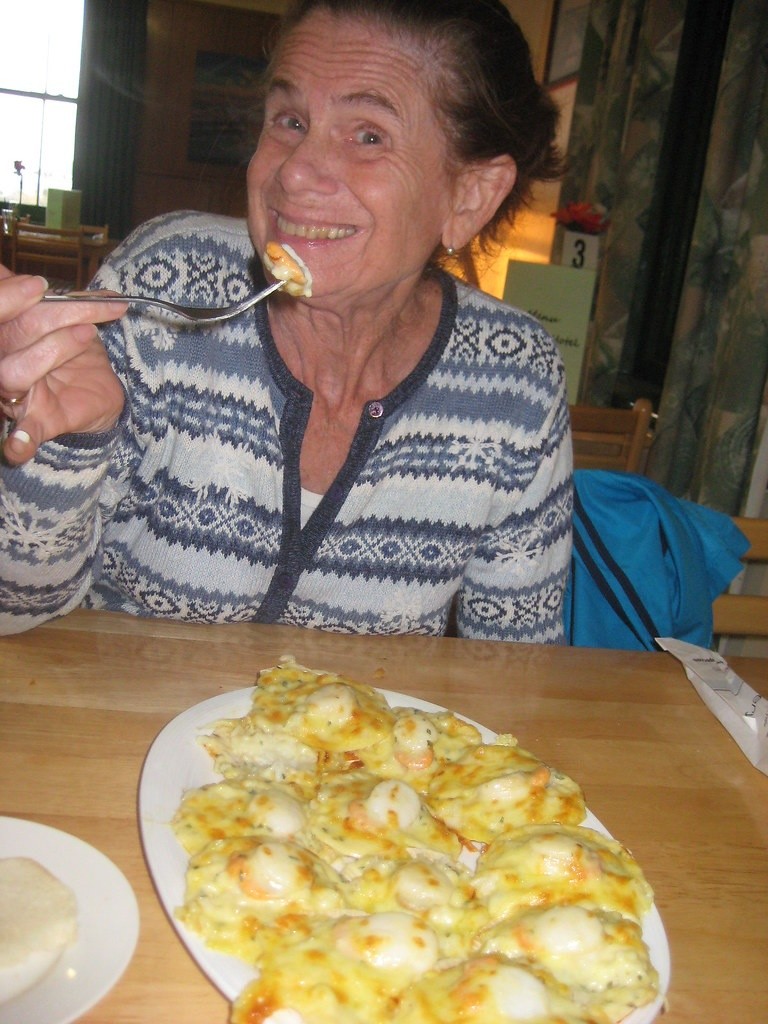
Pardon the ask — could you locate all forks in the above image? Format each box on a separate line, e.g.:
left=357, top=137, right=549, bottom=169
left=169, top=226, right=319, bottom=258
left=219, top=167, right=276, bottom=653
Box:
left=42, top=280, right=287, bottom=324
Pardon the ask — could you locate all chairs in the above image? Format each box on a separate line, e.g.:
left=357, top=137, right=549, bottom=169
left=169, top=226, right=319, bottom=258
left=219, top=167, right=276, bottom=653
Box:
left=567, top=398, right=768, bottom=657
left=11, top=219, right=109, bottom=292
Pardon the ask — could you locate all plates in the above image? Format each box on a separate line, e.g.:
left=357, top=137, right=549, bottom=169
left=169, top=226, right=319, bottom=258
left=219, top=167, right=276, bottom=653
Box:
left=1, top=816, right=139, bottom=1024
left=139, top=682, right=670, bottom=1024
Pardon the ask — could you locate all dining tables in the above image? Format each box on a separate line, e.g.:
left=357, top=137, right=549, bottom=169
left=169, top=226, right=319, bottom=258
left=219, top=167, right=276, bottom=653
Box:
left=0, top=608, right=768, bottom=1024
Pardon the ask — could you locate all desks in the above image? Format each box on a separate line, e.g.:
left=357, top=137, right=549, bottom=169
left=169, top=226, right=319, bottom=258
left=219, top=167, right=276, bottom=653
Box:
left=0, top=225, right=120, bottom=290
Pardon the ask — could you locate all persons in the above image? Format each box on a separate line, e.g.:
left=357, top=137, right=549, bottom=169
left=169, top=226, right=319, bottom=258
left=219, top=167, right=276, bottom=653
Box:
left=0, top=0, right=573, bottom=645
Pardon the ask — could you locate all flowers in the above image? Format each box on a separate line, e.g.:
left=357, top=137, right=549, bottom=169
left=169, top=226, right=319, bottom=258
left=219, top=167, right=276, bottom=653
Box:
left=13, top=160, right=25, bottom=211
left=550, top=201, right=613, bottom=235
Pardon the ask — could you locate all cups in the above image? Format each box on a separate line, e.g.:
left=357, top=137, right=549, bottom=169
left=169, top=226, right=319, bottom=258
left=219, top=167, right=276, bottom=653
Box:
left=2, top=209, right=15, bottom=235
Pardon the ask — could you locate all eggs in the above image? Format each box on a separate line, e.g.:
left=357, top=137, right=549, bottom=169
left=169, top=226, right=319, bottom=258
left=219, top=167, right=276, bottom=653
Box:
left=172, top=662, right=662, bottom=1023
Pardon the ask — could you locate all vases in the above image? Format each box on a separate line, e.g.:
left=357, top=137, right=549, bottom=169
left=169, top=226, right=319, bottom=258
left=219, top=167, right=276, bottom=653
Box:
left=563, top=227, right=600, bottom=268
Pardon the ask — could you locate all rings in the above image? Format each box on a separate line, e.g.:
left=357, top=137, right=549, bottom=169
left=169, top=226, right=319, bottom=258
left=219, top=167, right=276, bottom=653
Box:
left=1, top=397, right=23, bottom=407
